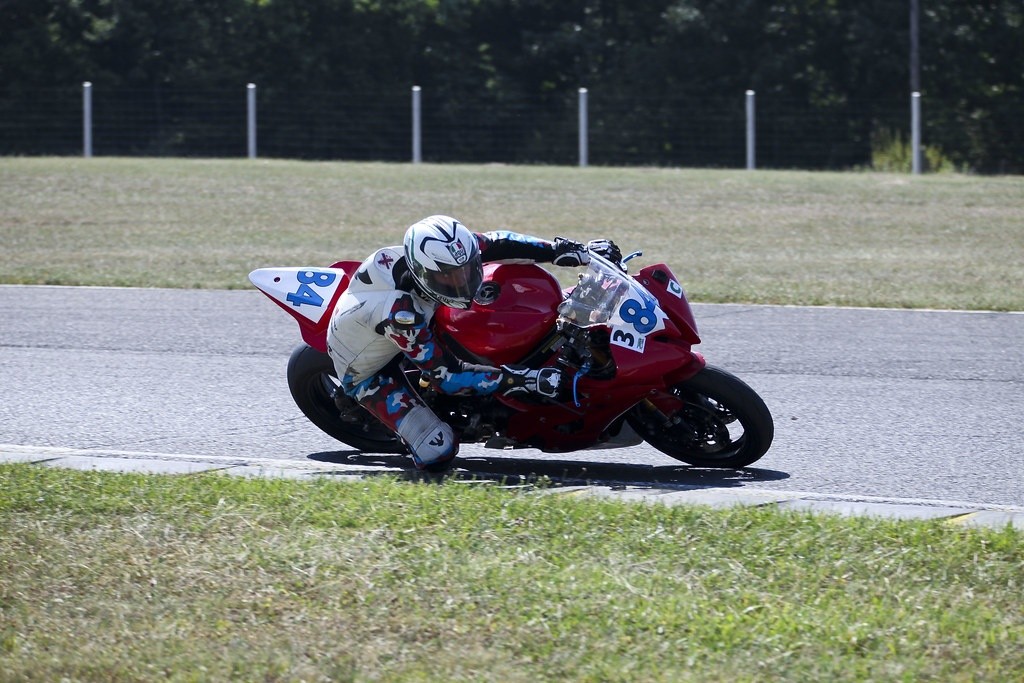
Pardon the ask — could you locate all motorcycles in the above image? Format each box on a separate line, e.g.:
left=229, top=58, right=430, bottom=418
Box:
left=247, top=250, right=775, bottom=471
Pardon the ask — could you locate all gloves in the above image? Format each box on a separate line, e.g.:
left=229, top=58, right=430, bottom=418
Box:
left=552, top=237, right=623, bottom=267
left=500, top=362, right=567, bottom=402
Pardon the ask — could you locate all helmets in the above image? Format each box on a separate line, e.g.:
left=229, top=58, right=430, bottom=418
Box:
left=402, top=215, right=484, bottom=310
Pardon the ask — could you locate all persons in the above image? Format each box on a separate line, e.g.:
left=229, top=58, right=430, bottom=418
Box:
left=326, top=214, right=623, bottom=477
